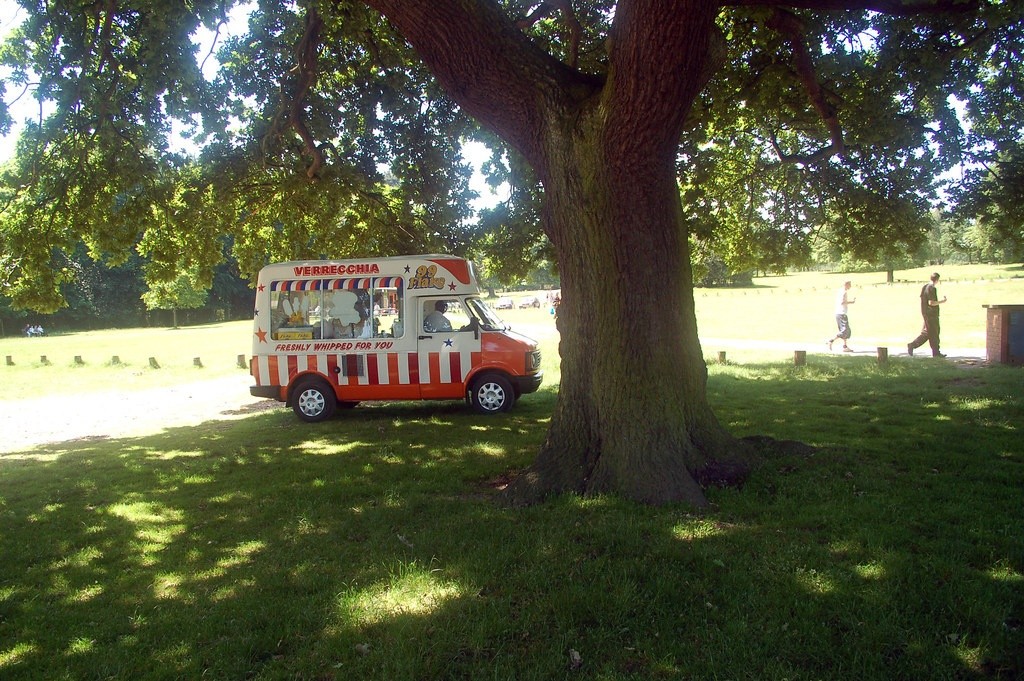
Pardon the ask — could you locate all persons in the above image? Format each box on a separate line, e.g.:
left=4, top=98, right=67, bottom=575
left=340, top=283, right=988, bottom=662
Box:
left=24, top=324, right=44, bottom=337
left=545, top=291, right=561, bottom=319
left=826, top=278, right=855, bottom=352
left=315, top=305, right=321, bottom=315
left=374, top=302, right=381, bottom=317
left=424, top=300, right=473, bottom=331
left=908, top=272, right=948, bottom=357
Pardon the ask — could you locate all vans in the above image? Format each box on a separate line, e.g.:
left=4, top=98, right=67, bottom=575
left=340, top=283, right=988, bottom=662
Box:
left=249, top=254, right=543, bottom=424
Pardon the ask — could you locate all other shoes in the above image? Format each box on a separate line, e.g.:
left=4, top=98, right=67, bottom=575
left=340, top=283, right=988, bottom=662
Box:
left=826, top=340, right=833, bottom=351
left=933, top=352, right=947, bottom=359
left=843, top=346, right=853, bottom=352
left=908, top=343, right=913, bottom=356
